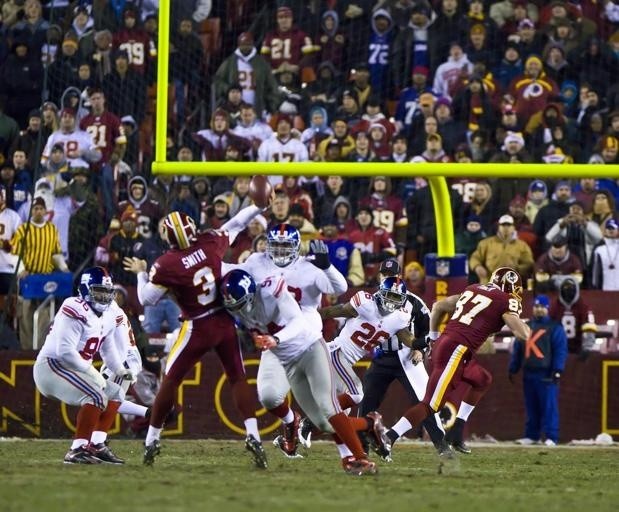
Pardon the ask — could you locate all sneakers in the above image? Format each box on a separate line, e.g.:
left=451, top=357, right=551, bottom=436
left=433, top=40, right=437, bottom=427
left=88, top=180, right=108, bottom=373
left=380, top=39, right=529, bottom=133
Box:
left=63, top=443, right=104, bottom=467
left=86, top=442, right=125, bottom=465
left=355, top=456, right=377, bottom=477
left=446, top=431, right=473, bottom=454
left=544, top=439, right=555, bottom=447
left=141, top=440, right=161, bottom=468
left=514, top=437, right=543, bottom=446
left=366, top=410, right=388, bottom=446
left=272, top=434, right=304, bottom=460
left=298, top=416, right=313, bottom=450
left=370, top=433, right=393, bottom=463
left=243, top=434, right=268, bottom=470
left=436, top=442, right=460, bottom=468
left=342, top=455, right=356, bottom=476
left=283, top=410, right=301, bottom=455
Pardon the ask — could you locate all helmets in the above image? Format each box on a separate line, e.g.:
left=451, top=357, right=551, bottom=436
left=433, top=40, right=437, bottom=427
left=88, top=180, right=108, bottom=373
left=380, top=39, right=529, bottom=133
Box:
left=76, top=266, right=116, bottom=312
left=218, top=268, right=257, bottom=321
left=378, top=277, right=407, bottom=314
left=489, top=265, right=523, bottom=306
left=156, top=210, right=199, bottom=251
left=266, top=223, right=302, bottom=270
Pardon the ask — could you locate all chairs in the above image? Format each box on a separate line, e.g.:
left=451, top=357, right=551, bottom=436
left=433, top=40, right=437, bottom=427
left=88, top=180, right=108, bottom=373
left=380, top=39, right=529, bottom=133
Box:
left=137, top=14, right=225, bottom=160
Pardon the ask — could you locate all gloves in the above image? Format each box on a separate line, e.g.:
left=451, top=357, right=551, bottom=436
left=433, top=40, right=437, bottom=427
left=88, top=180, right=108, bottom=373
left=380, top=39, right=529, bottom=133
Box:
left=88, top=365, right=106, bottom=389
left=508, top=369, right=516, bottom=384
left=252, top=334, right=278, bottom=351
left=119, top=366, right=136, bottom=385
left=304, top=238, right=329, bottom=270
left=552, top=370, right=560, bottom=385
left=425, top=331, right=439, bottom=357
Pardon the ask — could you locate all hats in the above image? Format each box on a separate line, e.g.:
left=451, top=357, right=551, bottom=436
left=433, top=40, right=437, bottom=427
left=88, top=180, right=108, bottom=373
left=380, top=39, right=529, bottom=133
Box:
left=0, top=1, right=619, bottom=231
left=377, top=258, right=401, bottom=274
left=531, top=294, right=550, bottom=311
left=113, top=283, right=128, bottom=301
